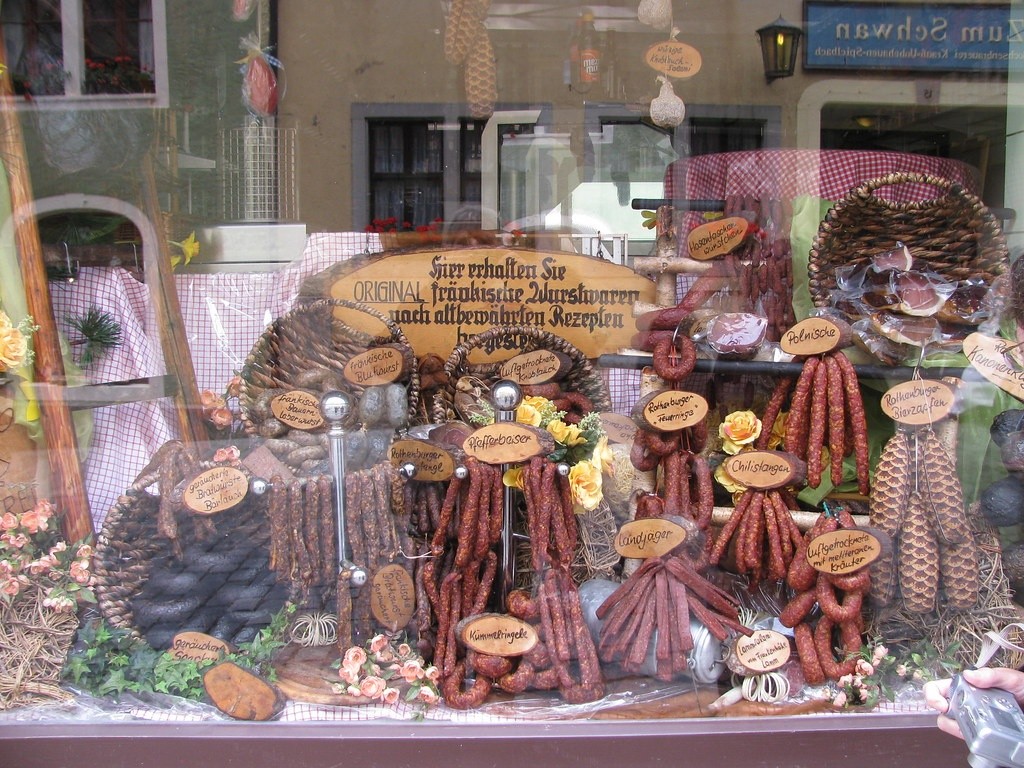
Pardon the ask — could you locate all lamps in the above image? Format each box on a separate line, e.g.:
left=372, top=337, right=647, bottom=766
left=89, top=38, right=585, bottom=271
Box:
left=754, top=15, right=804, bottom=84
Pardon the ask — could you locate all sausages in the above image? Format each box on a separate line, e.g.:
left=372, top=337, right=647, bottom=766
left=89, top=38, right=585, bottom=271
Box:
left=126, top=193, right=873, bottom=705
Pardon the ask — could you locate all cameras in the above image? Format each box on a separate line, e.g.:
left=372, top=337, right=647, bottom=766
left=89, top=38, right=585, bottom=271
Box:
left=945, top=673, right=1024, bottom=768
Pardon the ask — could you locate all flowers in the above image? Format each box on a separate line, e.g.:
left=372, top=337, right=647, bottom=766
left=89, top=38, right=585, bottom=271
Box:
left=829, top=639, right=935, bottom=709
left=168, top=232, right=200, bottom=268
left=466, top=388, right=616, bottom=514
left=640, top=211, right=658, bottom=232
left=1, top=307, right=37, bottom=383
left=719, top=408, right=844, bottom=502
left=21, top=56, right=156, bottom=100
left=333, top=629, right=446, bottom=711
left=0, top=501, right=97, bottom=615
left=364, top=217, right=444, bottom=236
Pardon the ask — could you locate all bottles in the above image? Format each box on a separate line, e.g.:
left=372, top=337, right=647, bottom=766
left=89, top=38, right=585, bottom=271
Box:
left=465, top=143, right=481, bottom=173
left=577, top=579, right=730, bottom=683
left=561, top=9, right=626, bottom=104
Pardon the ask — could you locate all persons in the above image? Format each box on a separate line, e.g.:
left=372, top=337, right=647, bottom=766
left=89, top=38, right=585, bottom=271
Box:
left=923, top=668, right=1024, bottom=741
